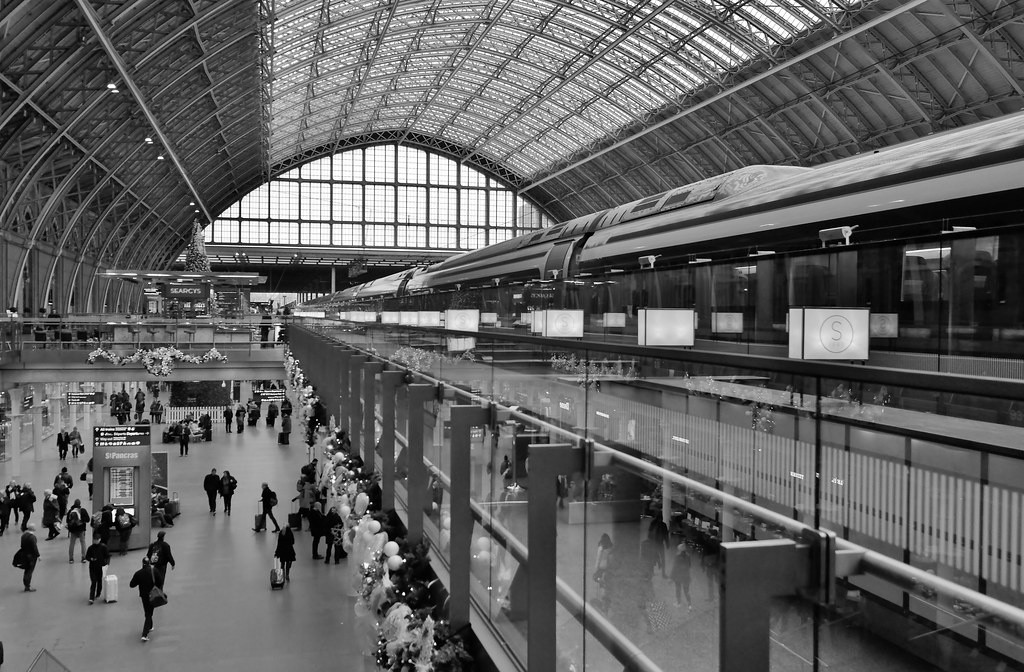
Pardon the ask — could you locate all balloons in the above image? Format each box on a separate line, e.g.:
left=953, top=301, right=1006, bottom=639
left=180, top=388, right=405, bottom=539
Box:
left=334, top=451, right=403, bottom=570
left=432, top=501, right=493, bottom=566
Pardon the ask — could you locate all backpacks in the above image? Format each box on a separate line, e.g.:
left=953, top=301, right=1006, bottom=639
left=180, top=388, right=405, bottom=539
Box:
left=68, top=508, right=84, bottom=526
left=90, top=510, right=111, bottom=528
left=150, top=542, right=166, bottom=565
left=118, top=513, right=131, bottom=528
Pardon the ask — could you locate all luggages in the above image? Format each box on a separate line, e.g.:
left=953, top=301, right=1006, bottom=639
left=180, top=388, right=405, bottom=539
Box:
left=287, top=501, right=300, bottom=529
left=255, top=500, right=267, bottom=531
left=278, top=432, right=285, bottom=443
left=165, top=491, right=180, bottom=518
left=270, top=555, right=286, bottom=590
left=104, top=575, right=119, bottom=604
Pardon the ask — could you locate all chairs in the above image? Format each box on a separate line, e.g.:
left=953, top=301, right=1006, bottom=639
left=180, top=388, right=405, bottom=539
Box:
left=632, top=250, right=997, bottom=315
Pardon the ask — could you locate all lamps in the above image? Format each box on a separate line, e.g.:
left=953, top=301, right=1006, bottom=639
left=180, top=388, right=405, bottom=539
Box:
left=189, top=200, right=195, bottom=206
left=111, top=89, right=121, bottom=93
left=106, top=70, right=116, bottom=88
left=144, top=131, right=153, bottom=142
left=148, top=141, right=154, bottom=145
left=195, top=209, right=200, bottom=213
left=157, top=153, right=165, bottom=160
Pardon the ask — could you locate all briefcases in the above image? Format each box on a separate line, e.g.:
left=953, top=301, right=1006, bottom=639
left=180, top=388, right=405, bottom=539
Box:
left=79, top=443, right=85, bottom=454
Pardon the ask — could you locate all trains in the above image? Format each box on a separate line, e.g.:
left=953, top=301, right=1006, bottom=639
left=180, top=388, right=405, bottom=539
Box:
left=295, top=109, right=1024, bottom=381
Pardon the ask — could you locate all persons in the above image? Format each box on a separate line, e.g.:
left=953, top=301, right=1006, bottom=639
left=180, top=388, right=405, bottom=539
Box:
left=0, top=457, right=93, bottom=541
left=500, top=454, right=513, bottom=492
left=115, top=506, right=136, bottom=556
left=274, top=523, right=295, bottom=580
left=69, top=426, right=83, bottom=458
left=95, top=504, right=115, bottom=556
left=66, top=499, right=90, bottom=563
left=204, top=468, right=220, bottom=516
left=48, top=308, right=61, bottom=328
left=219, top=470, right=237, bottom=516
left=291, top=458, right=344, bottom=564
left=594, top=512, right=725, bottom=633
left=557, top=473, right=572, bottom=509
left=130, top=556, right=163, bottom=641
left=252, top=482, right=280, bottom=532
left=110, top=380, right=292, bottom=458
left=146, top=531, right=175, bottom=592
left=21, top=522, right=42, bottom=591
left=151, top=485, right=170, bottom=528
left=86, top=534, right=110, bottom=603
left=57, top=427, right=70, bottom=460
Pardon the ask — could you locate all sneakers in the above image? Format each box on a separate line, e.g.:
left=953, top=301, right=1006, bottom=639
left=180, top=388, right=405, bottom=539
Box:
left=141, top=636, right=149, bottom=641
left=150, top=627, right=154, bottom=630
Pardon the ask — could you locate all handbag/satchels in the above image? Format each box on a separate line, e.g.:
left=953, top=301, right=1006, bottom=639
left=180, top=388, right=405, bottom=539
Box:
left=148, top=586, right=169, bottom=608
left=11, top=548, right=29, bottom=569
